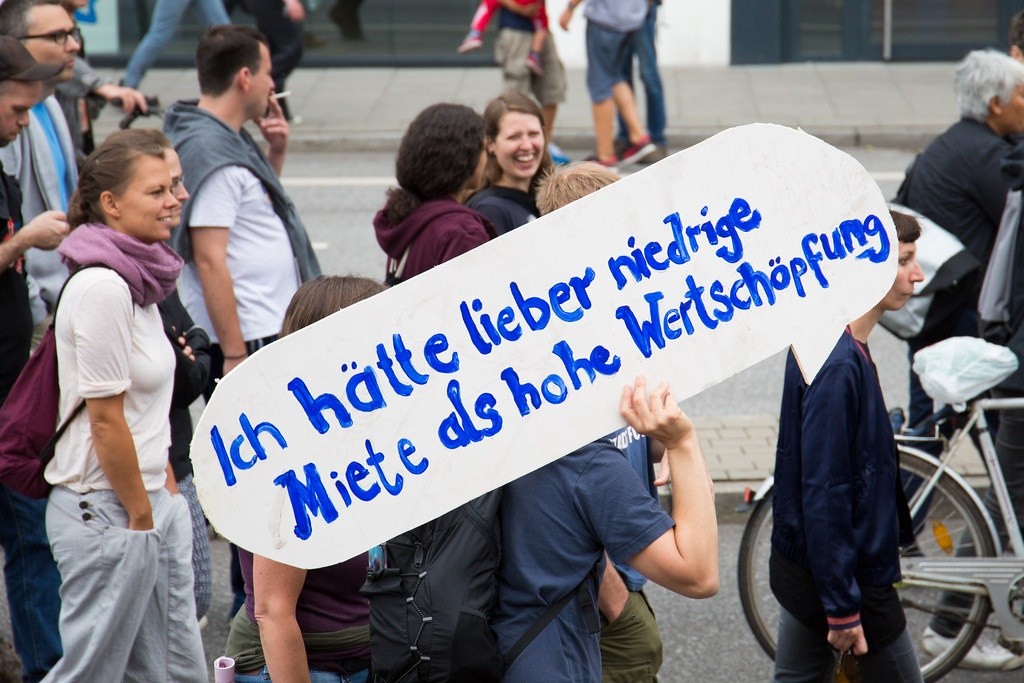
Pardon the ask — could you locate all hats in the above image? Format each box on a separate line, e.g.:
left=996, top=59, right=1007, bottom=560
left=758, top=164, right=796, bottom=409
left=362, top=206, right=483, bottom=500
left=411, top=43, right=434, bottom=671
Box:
left=0, top=35, right=64, bottom=80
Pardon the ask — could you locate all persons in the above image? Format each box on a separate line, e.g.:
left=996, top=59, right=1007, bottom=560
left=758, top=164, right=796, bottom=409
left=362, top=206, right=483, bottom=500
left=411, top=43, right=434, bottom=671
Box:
left=0, top=0, right=721, bottom=683
left=897, top=8, right=1024, bottom=671
left=769, top=211, right=923, bottom=683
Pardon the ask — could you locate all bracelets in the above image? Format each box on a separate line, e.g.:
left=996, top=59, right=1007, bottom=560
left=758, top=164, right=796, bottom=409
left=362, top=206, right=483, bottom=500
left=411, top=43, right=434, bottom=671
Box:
left=223, top=352, right=248, bottom=359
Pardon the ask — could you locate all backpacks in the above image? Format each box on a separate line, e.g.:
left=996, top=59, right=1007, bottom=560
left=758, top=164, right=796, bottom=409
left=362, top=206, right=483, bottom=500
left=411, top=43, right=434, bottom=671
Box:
left=0, top=261, right=134, bottom=499
left=365, top=480, right=605, bottom=683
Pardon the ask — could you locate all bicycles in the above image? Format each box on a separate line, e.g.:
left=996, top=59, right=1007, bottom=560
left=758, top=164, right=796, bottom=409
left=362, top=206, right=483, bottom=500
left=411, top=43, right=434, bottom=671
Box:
left=737, top=336, right=1024, bottom=683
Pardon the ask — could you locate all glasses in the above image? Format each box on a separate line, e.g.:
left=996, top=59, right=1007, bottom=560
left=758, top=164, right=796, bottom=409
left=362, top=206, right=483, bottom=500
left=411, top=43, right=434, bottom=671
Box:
left=16, top=27, right=80, bottom=44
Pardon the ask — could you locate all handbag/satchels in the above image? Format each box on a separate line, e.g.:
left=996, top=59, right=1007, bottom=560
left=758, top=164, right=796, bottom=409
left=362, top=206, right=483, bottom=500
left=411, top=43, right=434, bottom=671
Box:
left=881, top=198, right=982, bottom=341
left=978, top=190, right=1024, bottom=348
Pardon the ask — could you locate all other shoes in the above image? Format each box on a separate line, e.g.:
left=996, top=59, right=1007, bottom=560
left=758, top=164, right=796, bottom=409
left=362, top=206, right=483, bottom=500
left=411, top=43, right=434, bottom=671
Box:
left=637, top=141, right=668, bottom=164
left=583, top=154, right=618, bottom=173
left=616, top=132, right=656, bottom=168
left=547, top=143, right=572, bottom=167
left=526, top=51, right=545, bottom=78
left=919, top=624, right=1021, bottom=671
left=457, top=29, right=483, bottom=55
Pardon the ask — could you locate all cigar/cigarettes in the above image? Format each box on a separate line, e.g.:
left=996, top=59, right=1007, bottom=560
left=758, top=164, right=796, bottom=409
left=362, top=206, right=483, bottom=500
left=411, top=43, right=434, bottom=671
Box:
left=270, top=91, right=291, bottom=100
